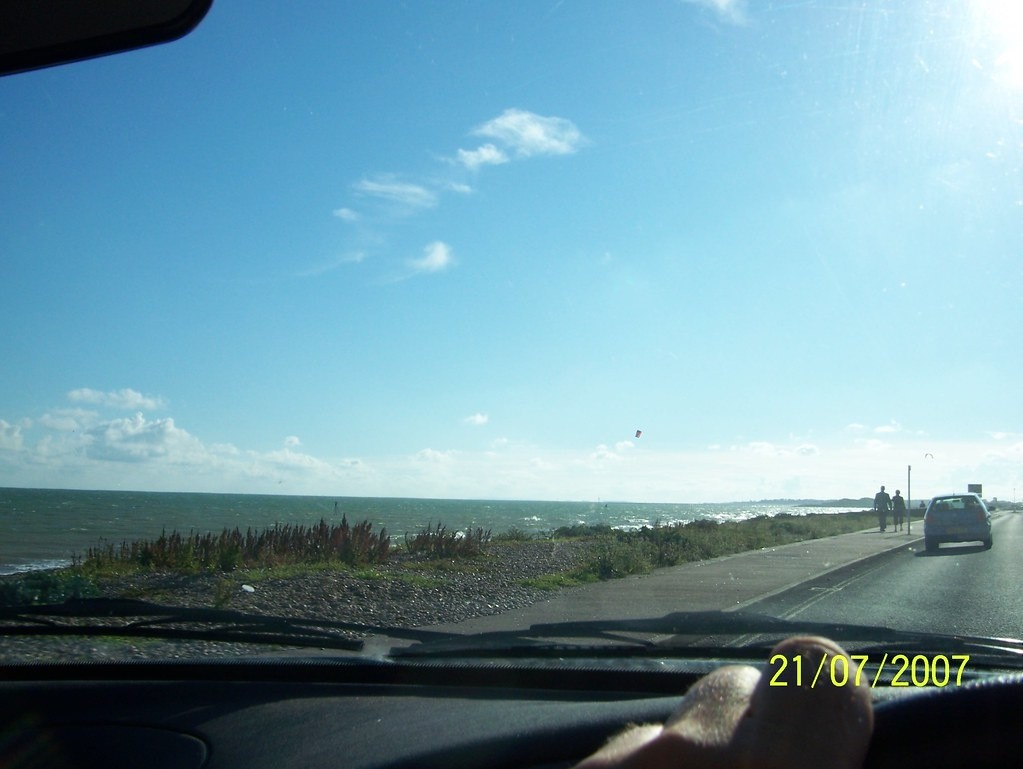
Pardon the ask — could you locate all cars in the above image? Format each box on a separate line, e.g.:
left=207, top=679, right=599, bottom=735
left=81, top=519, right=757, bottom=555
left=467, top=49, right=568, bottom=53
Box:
left=923, top=494, right=992, bottom=552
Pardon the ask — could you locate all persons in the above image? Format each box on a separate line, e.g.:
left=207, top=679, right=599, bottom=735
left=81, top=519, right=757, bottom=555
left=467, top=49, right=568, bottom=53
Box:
left=573, top=637, right=874, bottom=769
left=919, top=501, right=925, bottom=518
left=873, top=486, right=892, bottom=531
left=891, top=490, right=906, bottom=532
